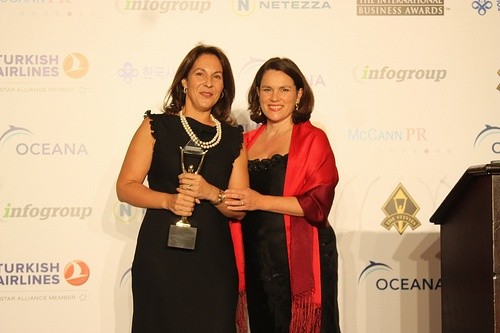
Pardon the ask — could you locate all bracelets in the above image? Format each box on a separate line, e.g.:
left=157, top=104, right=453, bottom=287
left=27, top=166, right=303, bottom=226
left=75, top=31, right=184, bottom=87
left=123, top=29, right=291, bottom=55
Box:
left=210, top=189, right=222, bottom=205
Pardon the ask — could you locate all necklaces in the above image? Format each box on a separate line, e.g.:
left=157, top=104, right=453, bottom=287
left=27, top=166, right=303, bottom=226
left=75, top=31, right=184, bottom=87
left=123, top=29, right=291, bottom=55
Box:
left=179, top=110, right=221, bottom=149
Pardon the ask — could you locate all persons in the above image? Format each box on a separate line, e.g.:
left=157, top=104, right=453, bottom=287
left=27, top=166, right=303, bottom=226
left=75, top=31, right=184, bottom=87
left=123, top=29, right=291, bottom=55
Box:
left=221, top=58, right=344, bottom=333
left=118, top=47, right=250, bottom=333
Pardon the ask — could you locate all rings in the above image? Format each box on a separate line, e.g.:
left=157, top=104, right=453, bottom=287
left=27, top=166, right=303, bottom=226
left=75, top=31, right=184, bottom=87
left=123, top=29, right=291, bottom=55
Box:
left=187, top=184, right=192, bottom=191
left=241, top=201, right=244, bottom=206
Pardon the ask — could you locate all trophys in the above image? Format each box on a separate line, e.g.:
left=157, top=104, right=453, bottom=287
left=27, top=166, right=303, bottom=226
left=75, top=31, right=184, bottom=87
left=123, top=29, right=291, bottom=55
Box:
left=176, top=147, right=208, bottom=227
